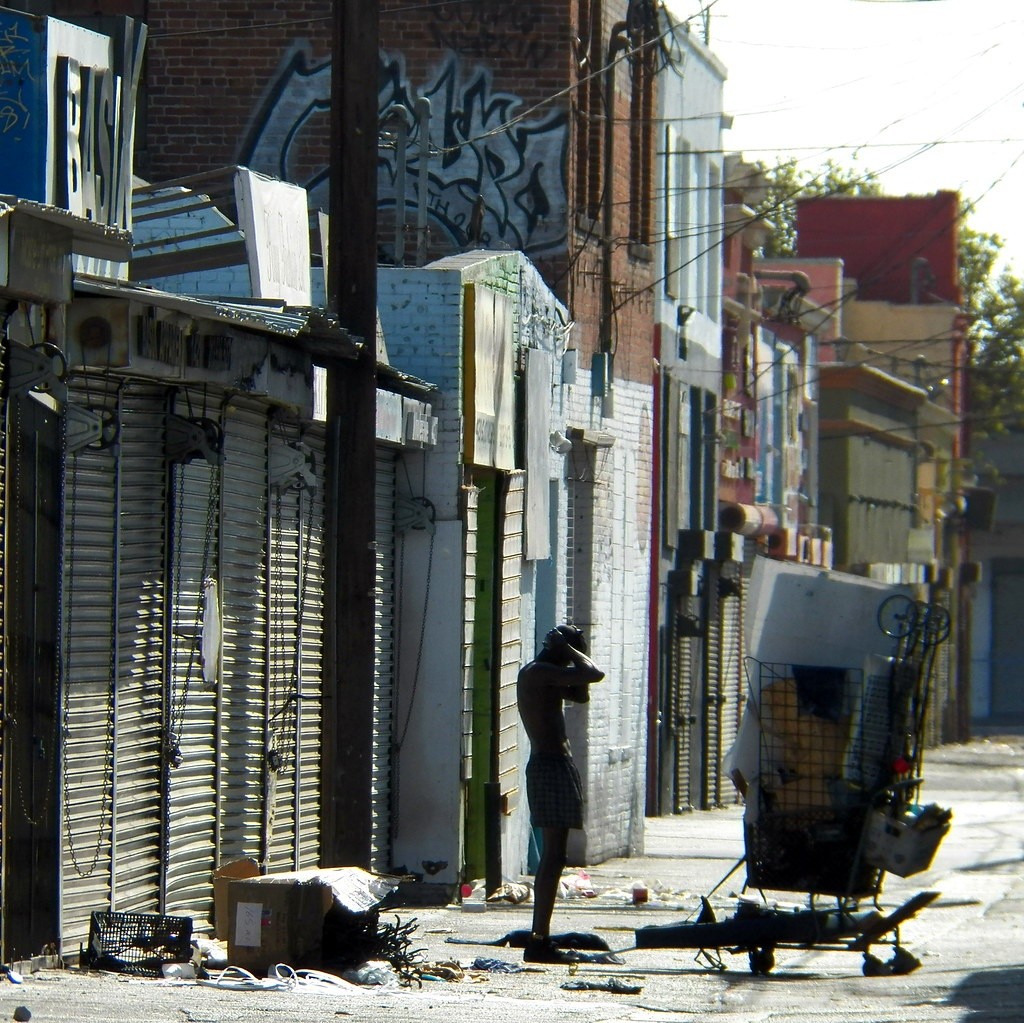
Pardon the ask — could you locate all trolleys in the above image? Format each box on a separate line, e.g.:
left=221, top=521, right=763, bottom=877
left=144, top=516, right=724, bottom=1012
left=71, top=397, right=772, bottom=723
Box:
left=742, top=662, right=925, bottom=914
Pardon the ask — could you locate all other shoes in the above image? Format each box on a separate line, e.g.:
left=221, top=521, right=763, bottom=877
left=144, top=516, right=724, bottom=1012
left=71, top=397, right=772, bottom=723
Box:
left=524, top=932, right=580, bottom=963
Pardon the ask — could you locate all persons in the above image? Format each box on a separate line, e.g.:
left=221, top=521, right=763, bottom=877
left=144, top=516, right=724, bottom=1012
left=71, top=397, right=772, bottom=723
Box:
left=517, top=627, right=607, bottom=964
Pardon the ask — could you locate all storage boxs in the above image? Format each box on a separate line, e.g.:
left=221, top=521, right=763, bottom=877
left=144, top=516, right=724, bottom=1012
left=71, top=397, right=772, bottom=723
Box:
left=865, top=801, right=951, bottom=878
left=88, top=910, right=192, bottom=979
left=211, top=858, right=404, bottom=980
